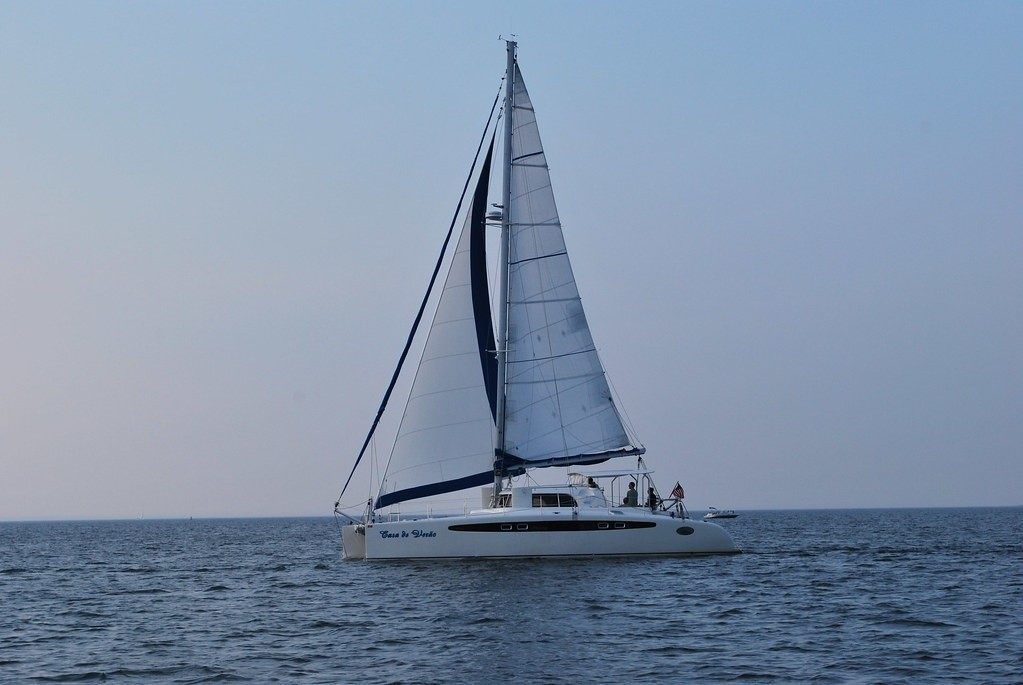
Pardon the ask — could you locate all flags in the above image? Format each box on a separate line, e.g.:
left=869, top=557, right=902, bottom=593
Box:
left=672, top=483, right=684, bottom=498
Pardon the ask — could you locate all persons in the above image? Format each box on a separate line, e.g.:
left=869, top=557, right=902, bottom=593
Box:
left=645, top=487, right=656, bottom=506
left=588, top=477, right=599, bottom=488
left=622, top=482, right=638, bottom=507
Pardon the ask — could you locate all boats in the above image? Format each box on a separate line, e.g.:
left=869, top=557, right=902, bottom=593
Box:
left=702, top=506, right=739, bottom=519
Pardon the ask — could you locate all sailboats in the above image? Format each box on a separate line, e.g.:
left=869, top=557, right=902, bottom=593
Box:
left=334, top=33, right=742, bottom=562
